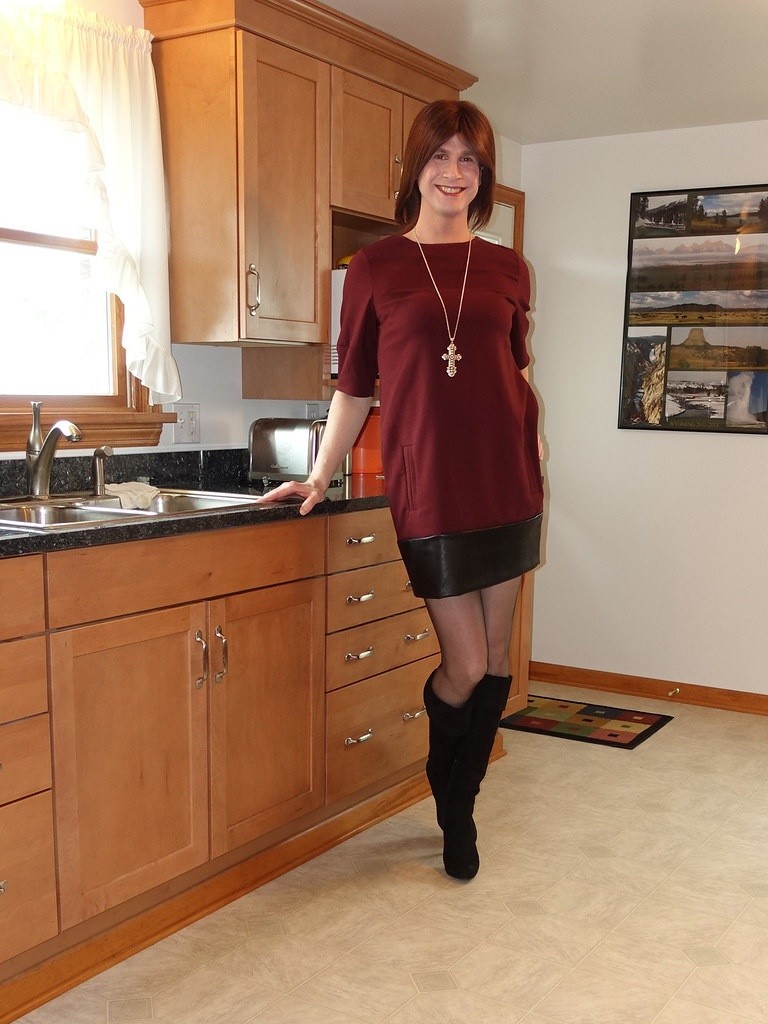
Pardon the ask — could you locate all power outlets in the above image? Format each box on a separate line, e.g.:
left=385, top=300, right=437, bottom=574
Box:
left=172, top=404, right=200, bottom=445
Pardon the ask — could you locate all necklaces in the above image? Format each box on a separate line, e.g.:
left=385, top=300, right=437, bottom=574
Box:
left=414, top=228, right=472, bottom=378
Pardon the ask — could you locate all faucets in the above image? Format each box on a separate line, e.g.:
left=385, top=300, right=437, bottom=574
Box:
left=27, top=401, right=83, bottom=502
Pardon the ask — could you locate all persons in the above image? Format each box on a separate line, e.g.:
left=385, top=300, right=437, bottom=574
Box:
left=254, top=98, right=545, bottom=881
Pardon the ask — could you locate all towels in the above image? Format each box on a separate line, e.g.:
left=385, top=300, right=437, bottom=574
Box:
left=104, top=482, right=160, bottom=509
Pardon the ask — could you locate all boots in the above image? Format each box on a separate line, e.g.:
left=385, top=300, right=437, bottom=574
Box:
left=423, top=667, right=513, bottom=881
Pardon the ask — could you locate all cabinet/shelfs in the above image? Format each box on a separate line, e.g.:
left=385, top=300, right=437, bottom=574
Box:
left=0, top=494, right=507, bottom=1024
left=137, top=0, right=525, bottom=401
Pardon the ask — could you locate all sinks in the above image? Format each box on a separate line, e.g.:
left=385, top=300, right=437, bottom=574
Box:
left=0, top=499, right=160, bottom=536
left=74, top=487, right=275, bottom=517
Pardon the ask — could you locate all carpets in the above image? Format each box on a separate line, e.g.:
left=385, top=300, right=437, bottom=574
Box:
left=499, top=695, right=674, bottom=750
